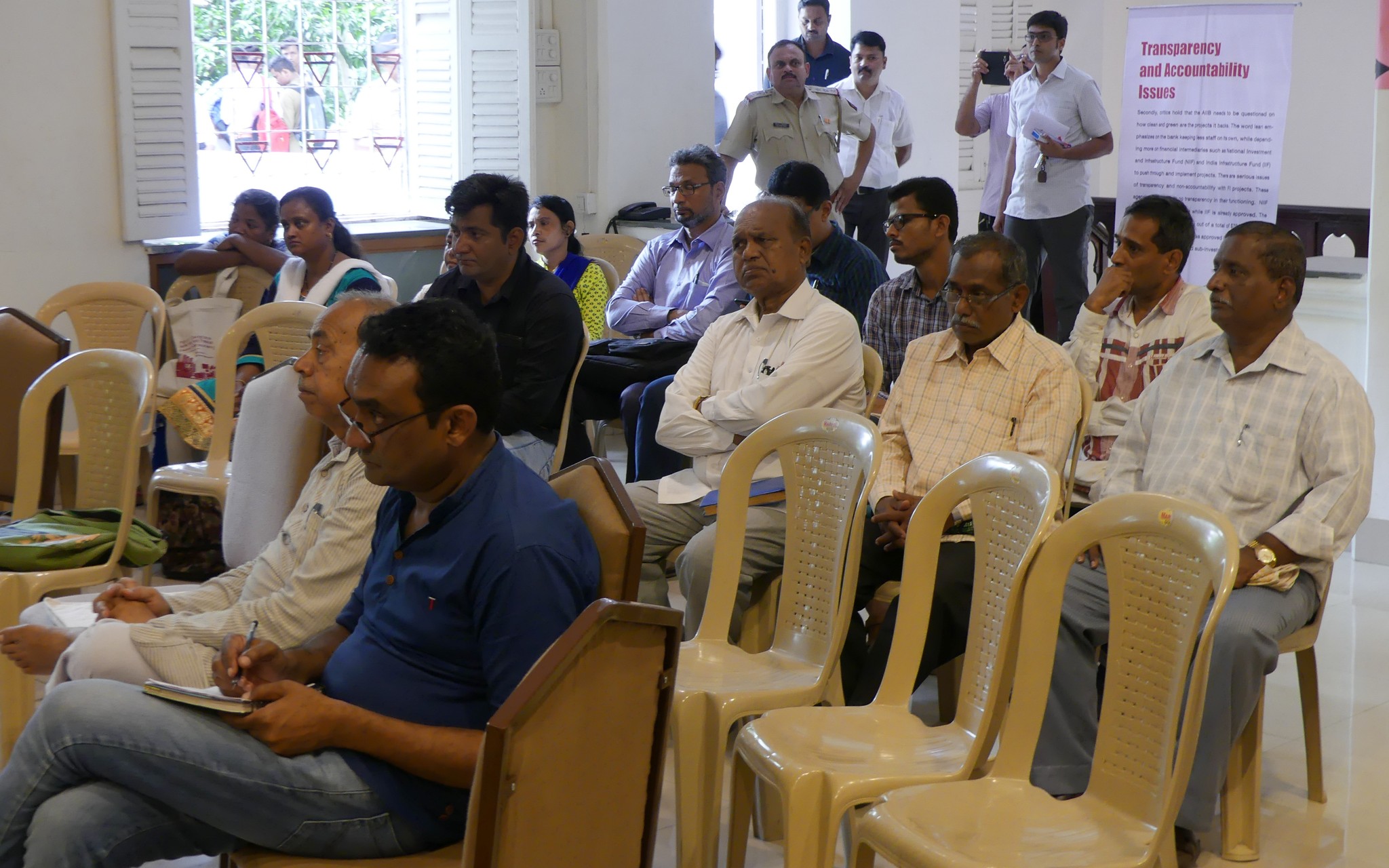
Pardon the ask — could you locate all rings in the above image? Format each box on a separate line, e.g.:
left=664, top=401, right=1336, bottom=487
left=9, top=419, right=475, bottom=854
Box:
left=975, top=54, right=979, bottom=58
left=974, top=63, right=977, bottom=67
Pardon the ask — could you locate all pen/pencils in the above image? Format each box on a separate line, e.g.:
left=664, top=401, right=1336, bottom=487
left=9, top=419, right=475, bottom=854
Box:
left=825, top=68, right=829, bottom=79
left=812, top=279, right=819, bottom=289
left=819, top=115, right=824, bottom=125
left=1237, top=424, right=1250, bottom=446
left=231, top=620, right=258, bottom=691
left=304, top=503, right=323, bottom=529
left=694, top=273, right=700, bottom=284
left=1010, top=417, right=1017, bottom=436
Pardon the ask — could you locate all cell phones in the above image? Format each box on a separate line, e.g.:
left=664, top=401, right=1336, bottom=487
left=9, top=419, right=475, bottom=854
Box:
left=980, top=51, right=1011, bottom=85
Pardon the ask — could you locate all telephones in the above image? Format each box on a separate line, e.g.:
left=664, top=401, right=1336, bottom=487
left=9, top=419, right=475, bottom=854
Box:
left=618, top=202, right=672, bottom=221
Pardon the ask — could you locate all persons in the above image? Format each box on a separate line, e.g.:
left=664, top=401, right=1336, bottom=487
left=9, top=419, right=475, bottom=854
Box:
left=762, top=0, right=851, bottom=88
left=161, top=186, right=389, bottom=582
left=0, top=296, right=603, bottom=868
left=715, top=42, right=728, bottom=144
left=419, top=139, right=1082, bottom=709
left=1030, top=221, right=1377, bottom=868
left=1061, top=193, right=1225, bottom=506
left=955, top=41, right=1035, bottom=234
left=1, top=290, right=398, bottom=695
left=992, top=10, right=1114, bottom=346
left=151, top=189, right=292, bottom=471
left=819, top=30, right=916, bottom=271
left=716, top=39, right=877, bottom=234
left=198, top=39, right=326, bottom=151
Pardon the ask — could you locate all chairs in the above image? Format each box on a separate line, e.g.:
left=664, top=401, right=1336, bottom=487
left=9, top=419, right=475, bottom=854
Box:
left=0, top=229, right=1334, bottom=868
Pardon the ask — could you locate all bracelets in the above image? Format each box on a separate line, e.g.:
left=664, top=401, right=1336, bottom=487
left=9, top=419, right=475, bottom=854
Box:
left=235, top=379, right=247, bottom=386
left=637, top=334, right=641, bottom=339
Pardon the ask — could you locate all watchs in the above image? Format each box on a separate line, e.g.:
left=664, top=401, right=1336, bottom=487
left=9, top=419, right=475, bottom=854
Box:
left=694, top=395, right=712, bottom=410
left=1247, top=538, right=1278, bottom=568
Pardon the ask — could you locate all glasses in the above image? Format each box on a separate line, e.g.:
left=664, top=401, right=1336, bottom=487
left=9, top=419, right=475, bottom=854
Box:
left=336, top=396, right=457, bottom=443
left=1025, top=33, right=1066, bottom=42
left=882, top=213, right=952, bottom=236
left=1015, top=53, right=1031, bottom=62
left=939, top=280, right=1028, bottom=309
left=661, top=179, right=717, bottom=197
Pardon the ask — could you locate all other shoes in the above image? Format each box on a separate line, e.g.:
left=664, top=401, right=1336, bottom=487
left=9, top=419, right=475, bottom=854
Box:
left=1174, top=826, right=1202, bottom=868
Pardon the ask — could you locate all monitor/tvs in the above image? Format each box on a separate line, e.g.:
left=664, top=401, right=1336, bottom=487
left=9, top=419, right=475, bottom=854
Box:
left=1276, top=204, right=1371, bottom=258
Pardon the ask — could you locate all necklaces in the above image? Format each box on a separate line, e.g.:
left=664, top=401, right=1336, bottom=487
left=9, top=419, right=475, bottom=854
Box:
left=305, top=250, right=337, bottom=297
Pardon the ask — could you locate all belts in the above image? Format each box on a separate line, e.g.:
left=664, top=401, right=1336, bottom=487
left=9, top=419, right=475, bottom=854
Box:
left=856, top=186, right=892, bottom=195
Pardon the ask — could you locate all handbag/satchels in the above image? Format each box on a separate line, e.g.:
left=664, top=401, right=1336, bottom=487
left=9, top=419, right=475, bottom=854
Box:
left=574, top=338, right=698, bottom=420
left=158, top=266, right=244, bottom=399
left=0, top=507, right=170, bottom=573
